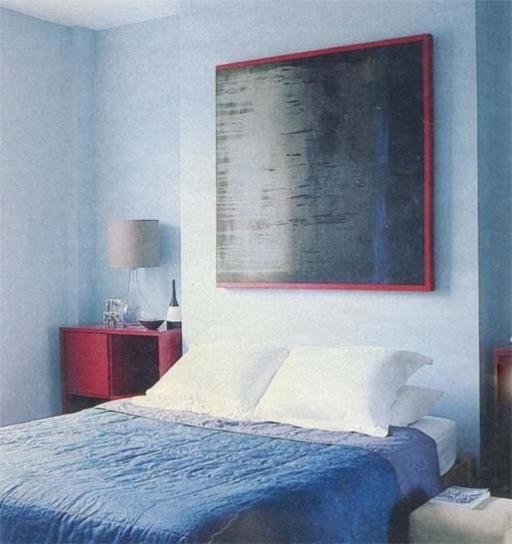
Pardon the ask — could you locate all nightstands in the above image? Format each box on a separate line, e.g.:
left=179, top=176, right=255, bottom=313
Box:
left=59, top=322, right=182, bottom=419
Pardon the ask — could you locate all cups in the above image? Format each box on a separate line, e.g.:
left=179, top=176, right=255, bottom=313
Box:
left=138, top=303, right=166, bottom=328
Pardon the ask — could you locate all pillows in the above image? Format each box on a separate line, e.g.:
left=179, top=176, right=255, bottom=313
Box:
left=390, top=385, right=444, bottom=427
left=249, top=345, right=434, bottom=437
left=146, top=342, right=289, bottom=422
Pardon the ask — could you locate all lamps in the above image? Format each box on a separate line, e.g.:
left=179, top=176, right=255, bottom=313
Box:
left=103, top=217, right=163, bottom=327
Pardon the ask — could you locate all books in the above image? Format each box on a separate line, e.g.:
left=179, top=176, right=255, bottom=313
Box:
left=429, top=485, right=492, bottom=511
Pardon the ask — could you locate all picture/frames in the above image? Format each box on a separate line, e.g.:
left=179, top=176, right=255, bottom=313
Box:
left=213, top=31, right=436, bottom=292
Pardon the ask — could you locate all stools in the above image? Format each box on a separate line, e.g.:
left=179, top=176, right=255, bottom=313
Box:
left=407, top=495, right=512, bottom=544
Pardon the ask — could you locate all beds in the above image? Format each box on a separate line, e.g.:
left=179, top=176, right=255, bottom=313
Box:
left=1, top=395, right=457, bottom=542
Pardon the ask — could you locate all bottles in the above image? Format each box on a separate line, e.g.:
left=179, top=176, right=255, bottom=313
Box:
left=166, top=279, right=182, bottom=328
